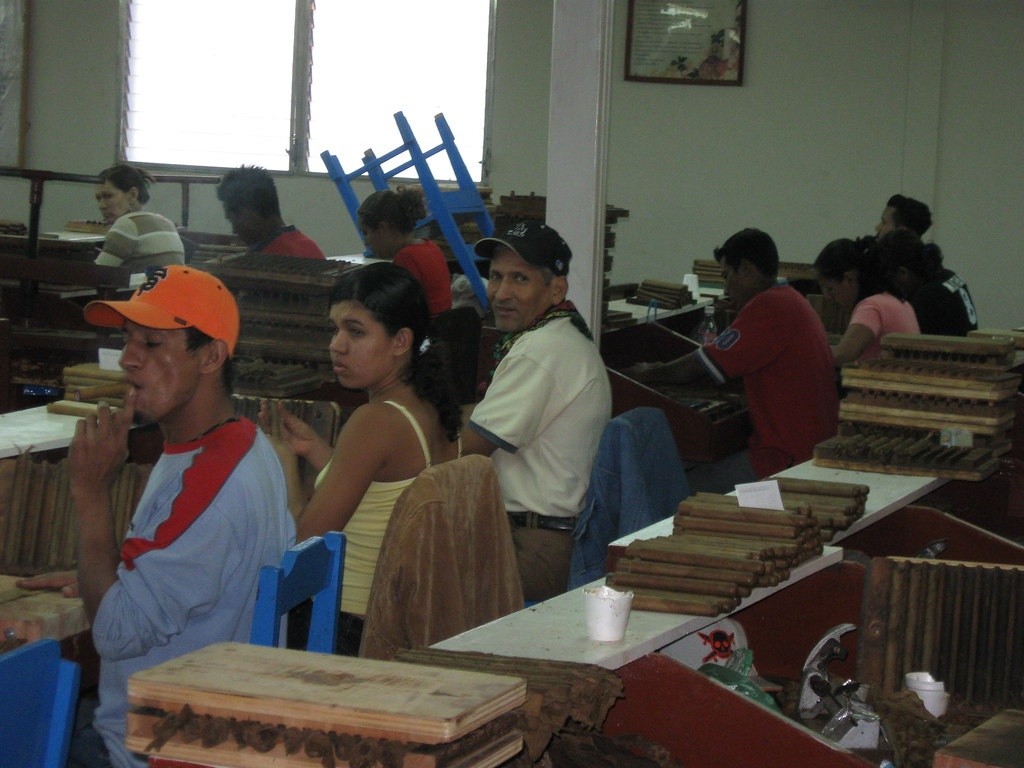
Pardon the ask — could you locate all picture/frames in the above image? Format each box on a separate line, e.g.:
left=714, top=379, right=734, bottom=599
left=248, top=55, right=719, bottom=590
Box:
left=625, top=0, right=748, bottom=86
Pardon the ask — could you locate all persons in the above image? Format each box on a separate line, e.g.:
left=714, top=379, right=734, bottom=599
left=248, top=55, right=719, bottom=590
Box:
left=95, top=164, right=185, bottom=288
left=257, top=262, right=463, bottom=657
left=217, top=168, right=325, bottom=259
left=357, top=188, right=452, bottom=320
left=879, top=228, right=978, bottom=336
left=620, top=228, right=840, bottom=494
left=812, top=235, right=921, bottom=366
left=15, top=264, right=297, bottom=768
left=875, top=194, right=932, bottom=244
left=463, top=218, right=613, bottom=602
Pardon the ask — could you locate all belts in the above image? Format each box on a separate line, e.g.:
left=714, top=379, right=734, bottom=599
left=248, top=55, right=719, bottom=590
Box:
left=513, top=513, right=573, bottom=532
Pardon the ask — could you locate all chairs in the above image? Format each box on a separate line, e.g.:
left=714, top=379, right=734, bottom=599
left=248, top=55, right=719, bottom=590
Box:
left=357, top=455, right=524, bottom=661
left=251, top=531, right=347, bottom=654
left=0, top=637, right=81, bottom=768
left=321, top=110, right=497, bottom=313
left=568, top=407, right=690, bottom=590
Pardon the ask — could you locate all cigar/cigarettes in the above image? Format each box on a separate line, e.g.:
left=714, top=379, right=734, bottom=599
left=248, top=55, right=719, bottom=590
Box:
left=74, top=383, right=127, bottom=402
left=626, top=279, right=692, bottom=310
left=883, top=562, right=1019, bottom=702
left=269, top=400, right=279, bottom=437
left=0, top=219, right=28, bottom=236
left=605, top=477, right=869, bottom=616
left=835, top=434, right=971, bottom=464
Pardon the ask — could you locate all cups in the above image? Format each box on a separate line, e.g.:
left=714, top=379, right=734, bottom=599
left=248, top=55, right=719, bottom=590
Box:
left=583, top=584, right=635, bottom=641
left=682, top=273, right=701, bottom=299
left=905, top=671, right=950, bottom=718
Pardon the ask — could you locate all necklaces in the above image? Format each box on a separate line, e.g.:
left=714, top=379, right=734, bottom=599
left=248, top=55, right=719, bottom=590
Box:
left=187, top=418, right=239, bottom=443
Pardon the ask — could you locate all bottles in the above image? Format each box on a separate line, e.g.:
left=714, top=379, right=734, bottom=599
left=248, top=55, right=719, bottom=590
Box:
left=697, top=306, right=717, bottom=345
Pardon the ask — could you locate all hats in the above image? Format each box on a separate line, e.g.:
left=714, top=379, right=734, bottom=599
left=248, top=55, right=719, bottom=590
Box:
left=473, top=220, right=572, bottom=275
left=84, top=264, right=239, bottom=360
